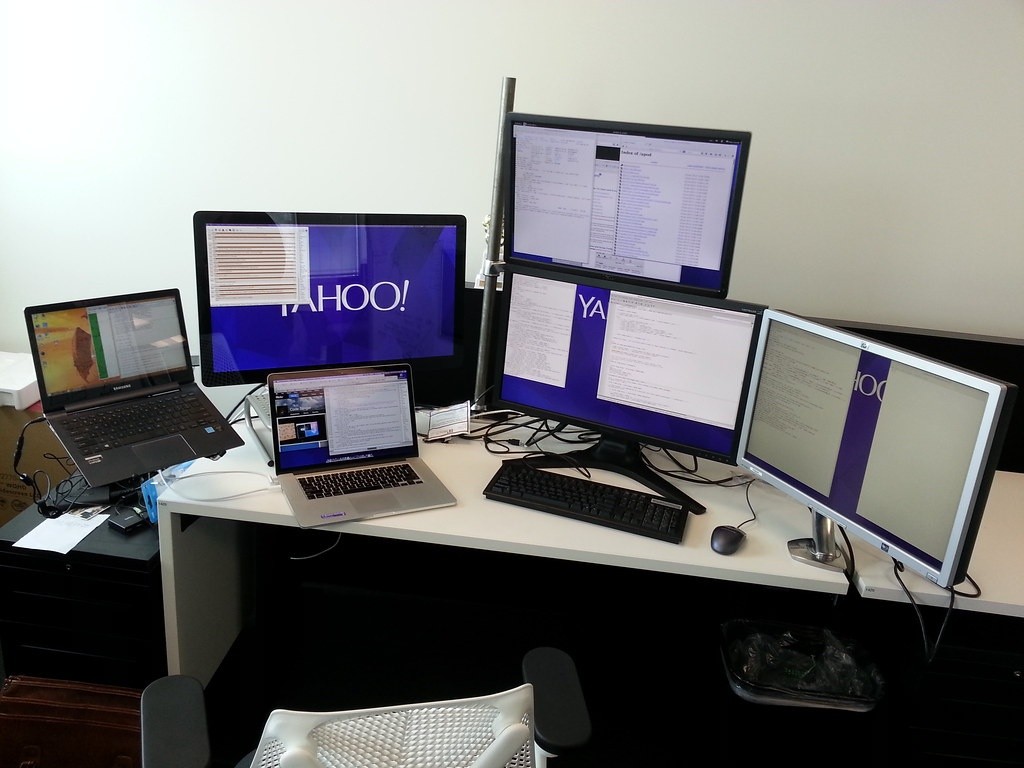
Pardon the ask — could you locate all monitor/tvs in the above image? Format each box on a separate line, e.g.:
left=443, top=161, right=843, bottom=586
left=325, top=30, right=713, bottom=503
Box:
left=194, top=211, right=467, bottom=386
left=504, top=112, right=752, bottom=298
left=736, top=308, right=1018, bottom=584
left=492, top=256, right=769, bottom=515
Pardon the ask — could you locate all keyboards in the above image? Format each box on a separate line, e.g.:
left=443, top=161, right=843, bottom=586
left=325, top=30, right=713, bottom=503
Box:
left=483, top=462, right=690, bottom=544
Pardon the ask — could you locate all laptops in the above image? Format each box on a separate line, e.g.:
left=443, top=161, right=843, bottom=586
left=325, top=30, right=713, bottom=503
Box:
left=23, top=288, right=246, bottom=488
left=267, top=364, right=458, bottom=528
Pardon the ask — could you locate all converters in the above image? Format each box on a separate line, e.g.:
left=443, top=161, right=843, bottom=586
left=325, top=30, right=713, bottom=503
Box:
left=54, top=487, right=110, bottom=511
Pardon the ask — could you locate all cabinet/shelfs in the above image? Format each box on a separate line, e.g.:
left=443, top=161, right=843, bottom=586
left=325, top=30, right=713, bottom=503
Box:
left=0, top=468, right=167, bottom=693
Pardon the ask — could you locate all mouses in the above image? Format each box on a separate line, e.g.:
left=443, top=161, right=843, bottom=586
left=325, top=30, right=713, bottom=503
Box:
left=710, top=526, right=746, bottom=555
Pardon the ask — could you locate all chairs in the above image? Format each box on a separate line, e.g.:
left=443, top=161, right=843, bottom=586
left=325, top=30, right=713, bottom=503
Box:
left=138, top=646, right=592, bottom=767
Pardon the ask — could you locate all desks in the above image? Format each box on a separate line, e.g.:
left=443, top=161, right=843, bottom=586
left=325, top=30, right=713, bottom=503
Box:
left=155, top=406, right=1024, bottom=696
